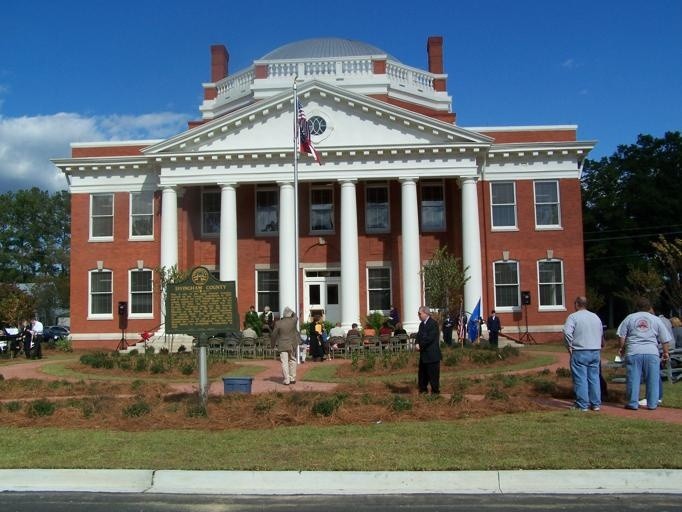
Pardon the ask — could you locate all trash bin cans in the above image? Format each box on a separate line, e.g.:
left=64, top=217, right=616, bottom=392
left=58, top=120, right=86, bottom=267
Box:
left=223, top=378, right=252, bottom=392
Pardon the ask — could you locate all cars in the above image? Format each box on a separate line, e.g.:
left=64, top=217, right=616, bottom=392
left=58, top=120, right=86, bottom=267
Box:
left=42, top=325, right=70, bottom=341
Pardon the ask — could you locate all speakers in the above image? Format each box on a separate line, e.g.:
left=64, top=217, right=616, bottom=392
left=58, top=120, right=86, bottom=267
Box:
left=118, top=302, right=127, bottom=329
left=521, top=291, right=530, bottom=305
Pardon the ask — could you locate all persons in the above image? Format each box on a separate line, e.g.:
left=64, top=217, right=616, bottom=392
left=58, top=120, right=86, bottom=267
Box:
left=598, top=306, right=608, bottom=405
left=257, top=304, right=273, bottom=326
left=242, top=323, right=259, bottom=358
left=389, top=304, right=398, bottom=326
left=22, top=320, right=30, bottom=358
left=561, top=294, right=603, bottom=411
left=226, top=328, right=242, bottom=355
left=308, top=313, right=409, bottom=361
left=270, top=306, right=301, bottom=385
left=443, top=312, right=502, bottom=347
left=617, top=295, right=681, bottom=409
left=415, top=307, right=442, bottom=393
left=243, top=304, right=259, bottom=326
left=29, top=318, right=44, bottom=359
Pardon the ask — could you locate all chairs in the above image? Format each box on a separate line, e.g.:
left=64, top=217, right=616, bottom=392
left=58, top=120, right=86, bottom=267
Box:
left=202, top=333, right=425, bottom=365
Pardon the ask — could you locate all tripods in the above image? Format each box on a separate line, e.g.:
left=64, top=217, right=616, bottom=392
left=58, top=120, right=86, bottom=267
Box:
left=116, top=329, right=129, bottom=351
left=520, top=305, right=537, bottom=344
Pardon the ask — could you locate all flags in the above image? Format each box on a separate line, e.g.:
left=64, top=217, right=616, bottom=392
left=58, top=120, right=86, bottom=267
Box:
left=457, top=303, right=463, bottom=341
left=295, top=102, right=320, bottom=162
left=468, top=298, right=481, bottom=342
